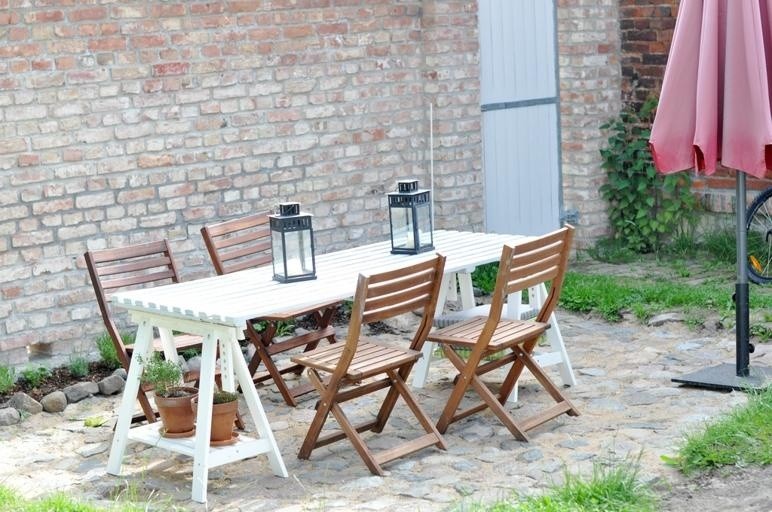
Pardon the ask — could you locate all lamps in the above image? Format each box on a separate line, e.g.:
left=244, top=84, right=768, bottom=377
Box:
left=268, top=179, right=434, bottom=283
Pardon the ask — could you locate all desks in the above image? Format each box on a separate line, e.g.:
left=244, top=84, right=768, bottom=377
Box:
left=105, top=230, right=577, bottom=503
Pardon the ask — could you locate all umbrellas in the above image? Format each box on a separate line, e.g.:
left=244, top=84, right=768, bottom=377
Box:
left=648, top=0, right=772, bottom=285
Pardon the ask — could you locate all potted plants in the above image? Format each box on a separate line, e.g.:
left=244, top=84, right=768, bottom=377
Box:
left=191, top=390, right=239, bottom=447
left=132, top=351, right=196, bottom=439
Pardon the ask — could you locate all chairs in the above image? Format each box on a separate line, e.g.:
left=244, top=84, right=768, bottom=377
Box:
left=83, top=237, right=246, bottom=430
left=425, top=222, right=581, bottom=444
left=200, top=209, right=363, bottom=407
left=289, top=252, right=450, bottom=479
